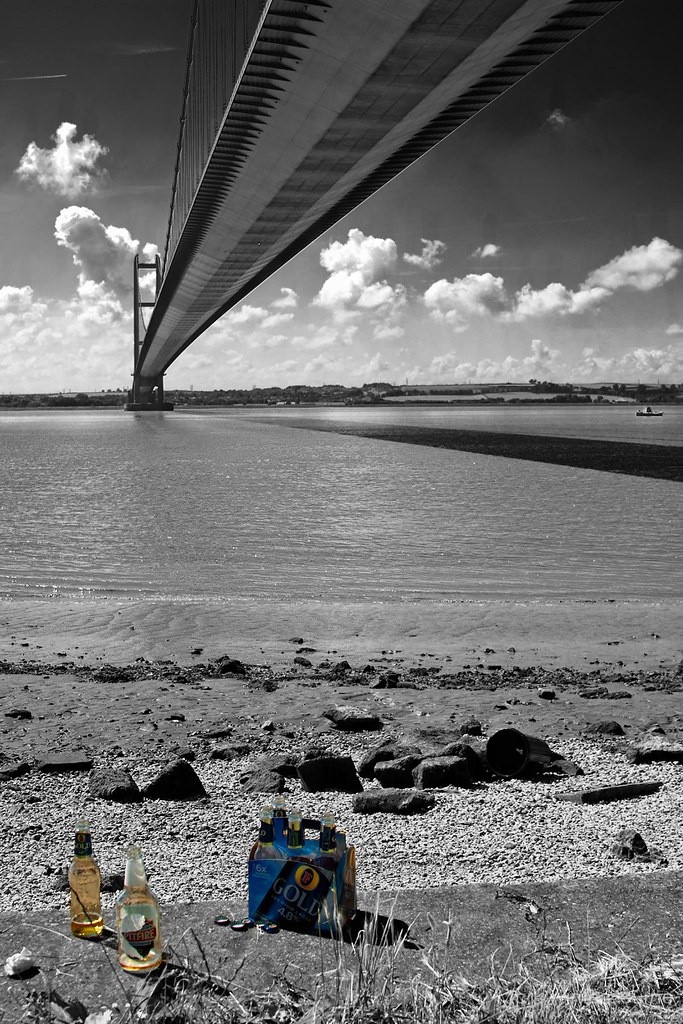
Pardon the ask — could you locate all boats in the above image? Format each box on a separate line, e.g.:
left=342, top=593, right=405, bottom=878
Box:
left=637, top=406, right=664, bottom=415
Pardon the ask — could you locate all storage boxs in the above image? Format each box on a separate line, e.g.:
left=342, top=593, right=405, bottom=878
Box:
left=247, top=838, right=356, bottom=934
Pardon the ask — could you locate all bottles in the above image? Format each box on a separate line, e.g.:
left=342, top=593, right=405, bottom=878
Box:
left=253, top=797, right=339, bottom=870
left=114, top=845, right=162, bottom=974
left=66, top=820, right=103, bottom=940
